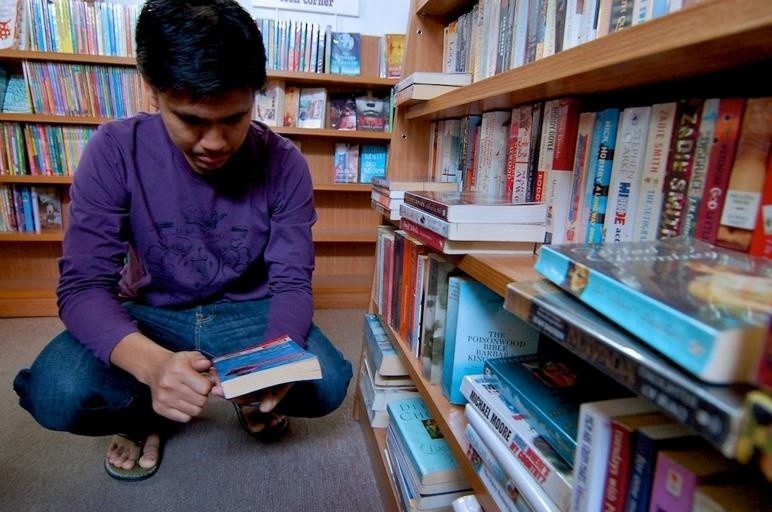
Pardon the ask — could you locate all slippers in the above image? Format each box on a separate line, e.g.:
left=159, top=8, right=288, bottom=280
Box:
left=104, top=431, right=162, bottom=481
left=232, top=401, right=292, bottom=443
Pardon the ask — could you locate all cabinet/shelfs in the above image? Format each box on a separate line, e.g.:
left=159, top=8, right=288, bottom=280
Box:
left=351, top=0, right=770, bottom=512
left=265, top=69, right=401, bottom=311
left=2, top=48, right=157, bottom=318
